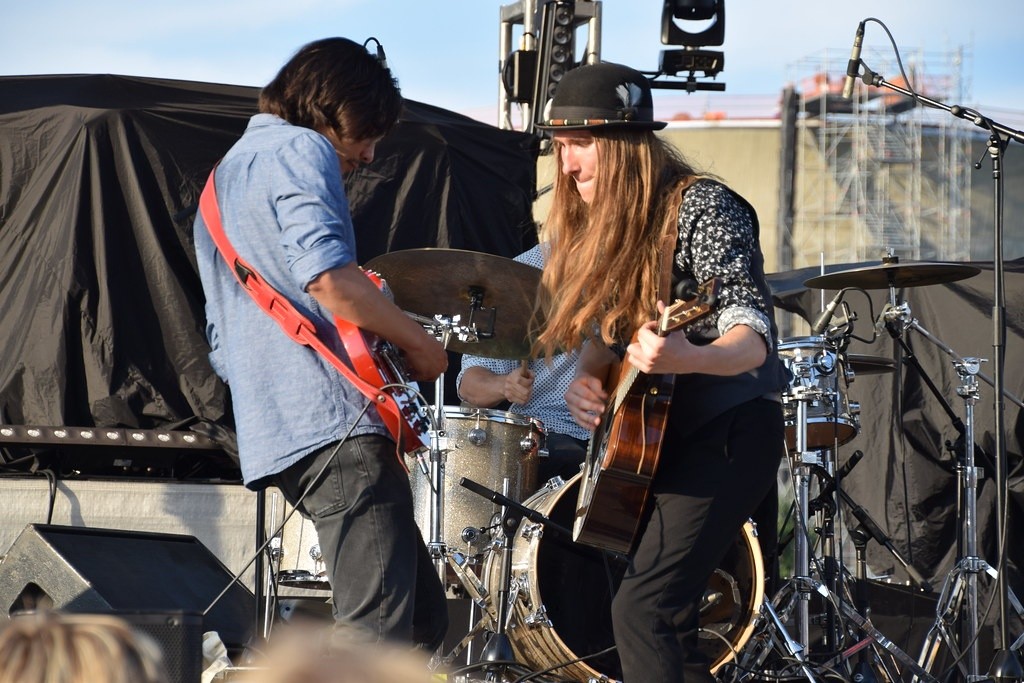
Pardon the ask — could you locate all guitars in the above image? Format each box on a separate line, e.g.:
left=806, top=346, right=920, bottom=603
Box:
left=572, top=282, right=716, bottom=553
left=200, top=167, right=431, bottom=455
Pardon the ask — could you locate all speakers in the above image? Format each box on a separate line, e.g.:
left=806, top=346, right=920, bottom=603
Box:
left=0, top=522, right=254, bottom=668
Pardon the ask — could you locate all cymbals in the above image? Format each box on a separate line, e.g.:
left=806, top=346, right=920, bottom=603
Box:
left=360, top=248, right=570, bottom=358
left=802, top=262, right=980, bottom=290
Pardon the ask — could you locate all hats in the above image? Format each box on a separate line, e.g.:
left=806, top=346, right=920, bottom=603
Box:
left=534, top=62, right=669, bottom=133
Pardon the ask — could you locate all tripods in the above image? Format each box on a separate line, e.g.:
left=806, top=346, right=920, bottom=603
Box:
left=425, top=315, right=486, bottom=620
left=450, top=477, right=631, bottom=683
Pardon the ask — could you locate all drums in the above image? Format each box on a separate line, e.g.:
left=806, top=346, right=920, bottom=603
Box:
left=772, top=340, right=858, bottom=450
left=274, top=408, right=764, bottom=683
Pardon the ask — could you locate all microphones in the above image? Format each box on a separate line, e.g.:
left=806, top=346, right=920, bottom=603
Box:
left=842, top=22, right=866, bottom=98
left=812, top=290, right=844, bottom=334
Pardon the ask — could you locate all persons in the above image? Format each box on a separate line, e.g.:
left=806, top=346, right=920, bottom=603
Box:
left=549, top=62, right=786, bottom=682
left=456, top=241, right=600, bottom=532
left=189, top=37, right=450, bottom=666
